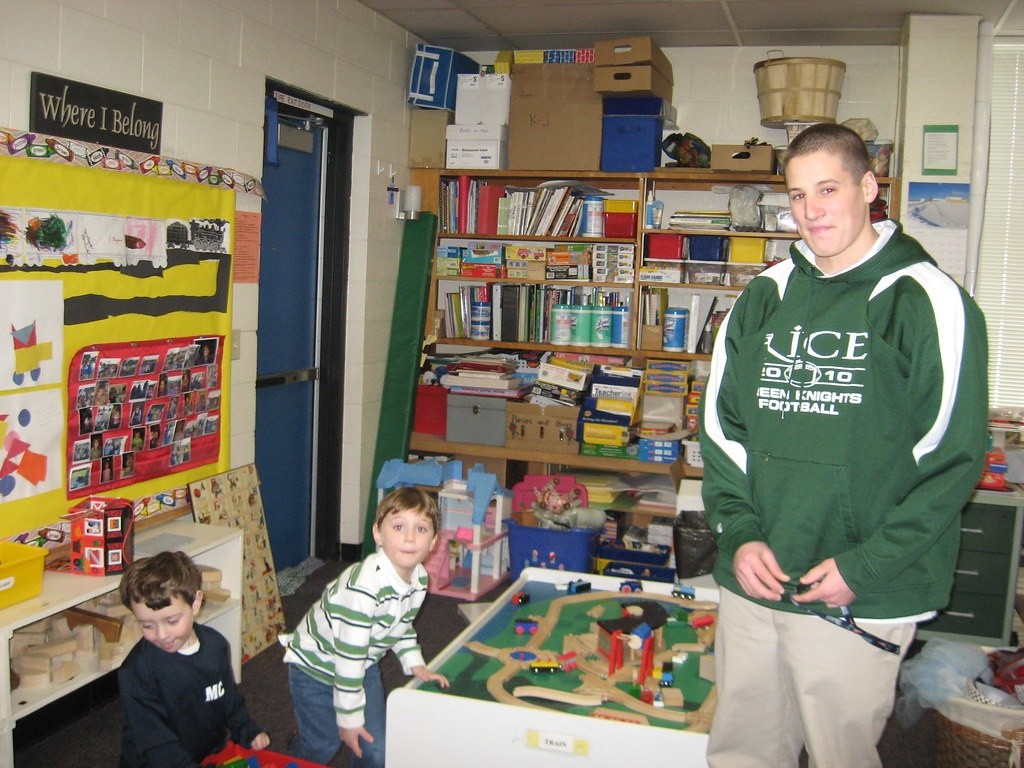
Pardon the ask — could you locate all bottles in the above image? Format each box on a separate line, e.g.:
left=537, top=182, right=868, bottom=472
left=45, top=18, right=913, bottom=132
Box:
left=663, top=307, right=687, bottom=353
left=581, top=195, right=604, bottom=239
left=549, top=304, right=630, bottom=348
left=471, top=302, right=491, bottom=341
left=646, top=190, right=663, bottom=230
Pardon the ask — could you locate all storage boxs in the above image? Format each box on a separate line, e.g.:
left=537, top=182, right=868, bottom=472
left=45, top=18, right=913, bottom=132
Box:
left=409, top=34, right=773, bottom=287
left=0, top=542, right=48, bottom=609
left=640, top=326, right=665, bottom=351
left=503, top=521, right=592, bottom=582
left=946, top=698, right=1024, bottom=744
left=410, top=358, right=703, bottom=469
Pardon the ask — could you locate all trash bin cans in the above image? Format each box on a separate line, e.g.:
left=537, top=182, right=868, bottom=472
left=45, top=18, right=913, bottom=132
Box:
left=672, top=509, right=720, bottom=581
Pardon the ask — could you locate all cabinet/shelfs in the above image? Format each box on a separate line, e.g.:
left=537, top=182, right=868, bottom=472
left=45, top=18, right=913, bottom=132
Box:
left=0, top=525, right=243, bottom=731
left=913, top=482, right=1022, bottom=646
left=402, top=171, right=793, bottom=476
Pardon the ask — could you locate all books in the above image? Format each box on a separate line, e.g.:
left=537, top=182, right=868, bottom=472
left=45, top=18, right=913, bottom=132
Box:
left=438, top=175, right=583, bottom=237
left=441, top=358, right=533, bottom=399
left=637, top=285, right=669, bottom=351
left=444, top=282, right=620, bottom=348
left=667, top=210, right=731, bottom=231
left=687, top=293, right=700, bottom=353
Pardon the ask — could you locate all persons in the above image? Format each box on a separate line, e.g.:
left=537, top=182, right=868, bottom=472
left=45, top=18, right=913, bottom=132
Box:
left=284, top=487, right=450, bottom=768
left=699, top=122, right=989, bottom=768
left=73, top=344, right=215, bottom=484
left=117, top=550, right=270, bottom=768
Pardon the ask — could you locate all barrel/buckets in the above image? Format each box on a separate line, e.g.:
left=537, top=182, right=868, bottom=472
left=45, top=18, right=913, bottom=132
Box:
left=753, top=49, right=846, bottom=127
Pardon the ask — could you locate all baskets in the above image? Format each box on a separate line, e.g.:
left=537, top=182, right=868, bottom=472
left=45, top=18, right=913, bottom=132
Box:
left=932, top=696, right=1024, bottom=768
